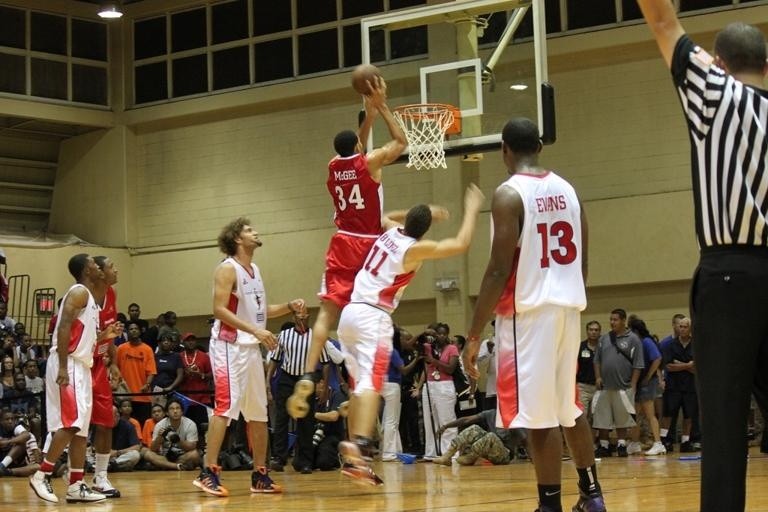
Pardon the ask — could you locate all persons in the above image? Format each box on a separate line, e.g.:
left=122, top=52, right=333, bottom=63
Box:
left=31, top=254, right=121, bottom=504
left=0, top=276, right=702, bottom=475
left=192, top=216, right=305, bottom=495
left=336, top=184, right=485, bottom=487
left=287, top=75, right=407, bottom=417
left=462, top=117, right=605, bottom=511
left=640, top=0, right=768, bottom=512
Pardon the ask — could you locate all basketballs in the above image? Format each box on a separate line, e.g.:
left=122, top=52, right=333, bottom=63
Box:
left=351, top=65, right=381, bottom=95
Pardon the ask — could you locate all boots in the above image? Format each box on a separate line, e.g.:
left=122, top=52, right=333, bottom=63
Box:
left=432, top=445, right=479, bottom=466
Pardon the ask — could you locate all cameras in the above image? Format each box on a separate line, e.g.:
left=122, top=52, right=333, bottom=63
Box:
left=312, top=424, right=325, bottom=445
left=163, top=429, right=180, bottom=443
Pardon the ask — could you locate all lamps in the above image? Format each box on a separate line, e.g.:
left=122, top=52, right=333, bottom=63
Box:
left=96, top=0, right=124, bottom=20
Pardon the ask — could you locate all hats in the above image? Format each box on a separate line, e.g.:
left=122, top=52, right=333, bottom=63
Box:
left=182, top=332, right=194, bottom=343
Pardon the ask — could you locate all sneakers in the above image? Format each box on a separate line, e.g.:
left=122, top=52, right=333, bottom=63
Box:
left=594, top=442, right=700, bottom=457
left=338, top=437, right=384, bottom=487
left=249, top=467, right=281, bottom=494
left=286, top=376, right=314, bottom=419
left=192, top=465, right=229, bottom=497
left=28, top=471, right=119, bottom=504
